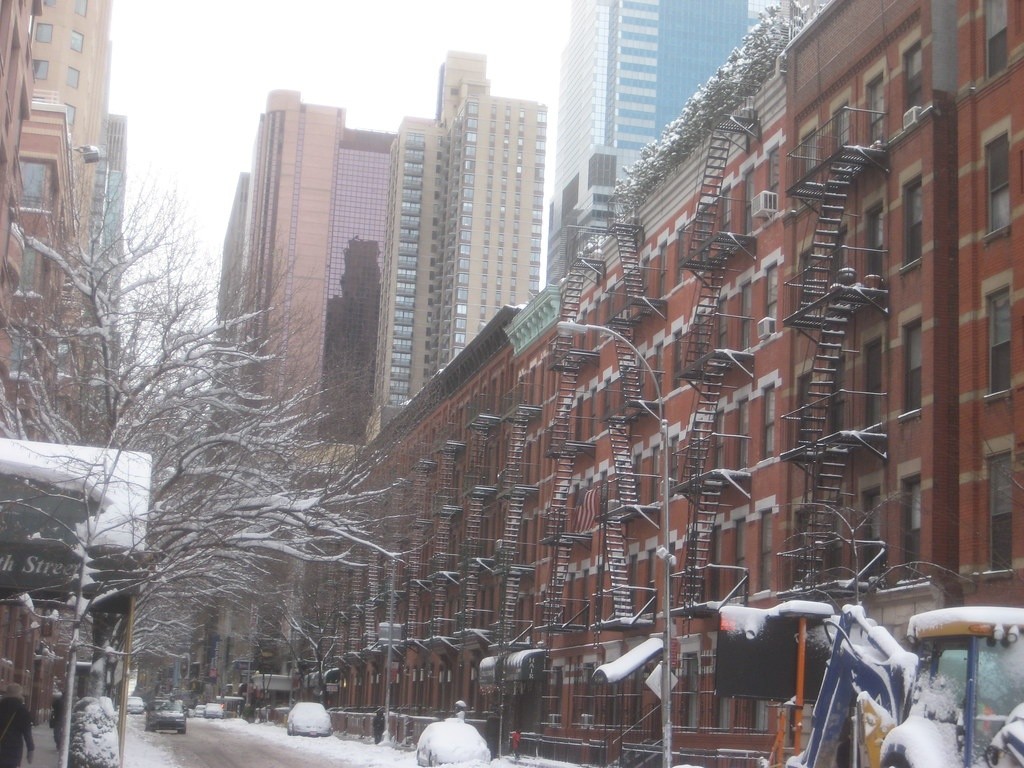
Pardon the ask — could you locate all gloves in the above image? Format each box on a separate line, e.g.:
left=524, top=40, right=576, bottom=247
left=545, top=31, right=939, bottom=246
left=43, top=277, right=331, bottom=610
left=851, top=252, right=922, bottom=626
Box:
left=27, top=751, right=33, bottom=764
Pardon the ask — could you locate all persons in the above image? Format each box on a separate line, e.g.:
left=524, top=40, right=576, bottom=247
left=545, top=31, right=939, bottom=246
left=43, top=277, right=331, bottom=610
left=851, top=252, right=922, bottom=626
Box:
left=512, top=728, right=521, bottom=759
left=486, top=711, right=500, bottom=758
left=52, top=693, right=66, bottom=750
left=372, top=708, right=386, bottom=744
left=0, top=682, right=34, bottom=768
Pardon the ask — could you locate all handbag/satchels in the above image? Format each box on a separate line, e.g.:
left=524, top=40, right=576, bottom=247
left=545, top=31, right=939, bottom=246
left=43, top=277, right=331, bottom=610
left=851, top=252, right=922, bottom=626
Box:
left=49, top=715, right=55, bottom=727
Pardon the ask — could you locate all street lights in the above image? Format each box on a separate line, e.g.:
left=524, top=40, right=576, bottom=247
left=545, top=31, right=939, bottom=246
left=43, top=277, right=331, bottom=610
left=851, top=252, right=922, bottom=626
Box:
left=555, top=320, right=673, bottom=768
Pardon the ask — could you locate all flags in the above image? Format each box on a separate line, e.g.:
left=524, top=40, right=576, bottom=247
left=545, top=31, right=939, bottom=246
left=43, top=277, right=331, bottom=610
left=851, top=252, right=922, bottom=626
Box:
left=573, top=487, right=596, bottom=534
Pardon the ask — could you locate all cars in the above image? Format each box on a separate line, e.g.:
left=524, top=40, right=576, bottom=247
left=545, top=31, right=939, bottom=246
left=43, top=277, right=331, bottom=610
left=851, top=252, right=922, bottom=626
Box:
left=287, top=702, right=333, bottom=737
left=203, top=702, right=223, bottom=719
left=144, top=699, right=188, bottom=734
left=415, top=720, right=491, bottom=765
left=188, top=709, right=194, bottom=717
left=195, top=705, right=205, bottom=717
left=127, top=697, right=145, bottom=714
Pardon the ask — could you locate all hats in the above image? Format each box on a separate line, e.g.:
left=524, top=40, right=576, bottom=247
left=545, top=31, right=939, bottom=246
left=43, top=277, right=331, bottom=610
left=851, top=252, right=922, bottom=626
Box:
left=7, top=683, right=23, bottom=697
